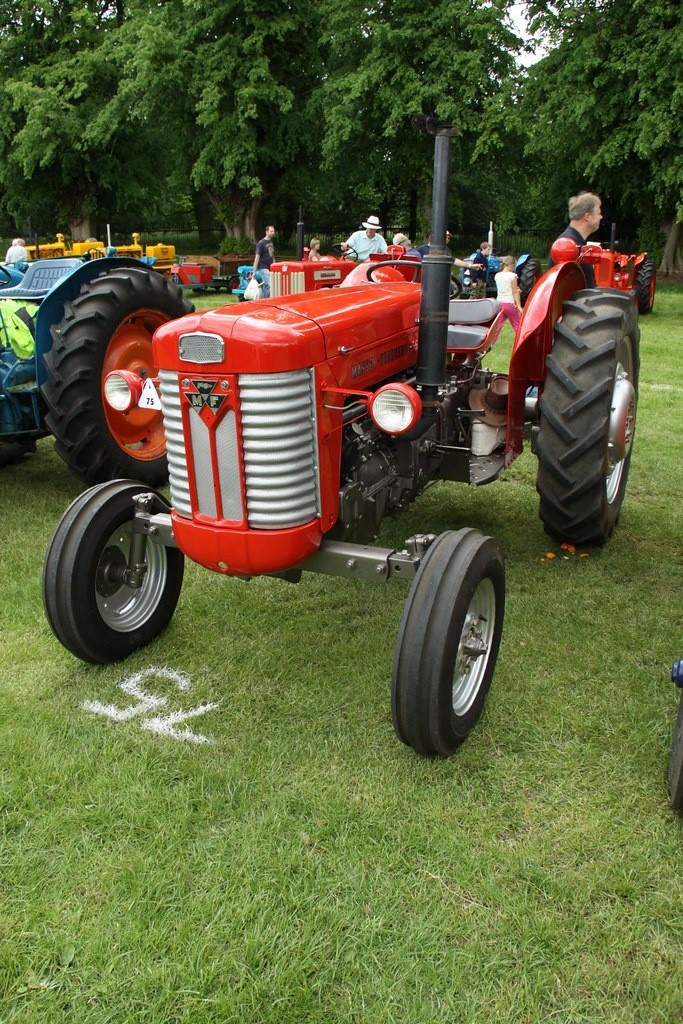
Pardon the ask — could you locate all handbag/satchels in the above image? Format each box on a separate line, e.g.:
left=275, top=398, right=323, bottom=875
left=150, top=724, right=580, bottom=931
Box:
left=243, top=278, right=262, bottom=300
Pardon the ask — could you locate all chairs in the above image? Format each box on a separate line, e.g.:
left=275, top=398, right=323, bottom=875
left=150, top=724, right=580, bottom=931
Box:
left=447, top=297, right=513, bottom=353
left=0, top=258, right=83, bottom=300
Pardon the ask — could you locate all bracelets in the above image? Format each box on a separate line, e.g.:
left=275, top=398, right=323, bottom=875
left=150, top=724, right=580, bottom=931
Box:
left=469, top=263, right=472, bottom=269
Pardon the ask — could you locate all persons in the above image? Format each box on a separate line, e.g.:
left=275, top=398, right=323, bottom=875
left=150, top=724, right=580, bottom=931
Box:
left=393, top=233, right=422, bottom=285
left=417, top=228, right=483, bottom=289
left=252, top=226, right=276, bottom=299
left=308, top=237, right=322, bottom=263
left=5, top=238, right=28, bottom=264
left=547, top=191, right=610, bottom=288
left=489, top=254, right=523, bottom=346
left=471, top=241, right=491, bottom=298
left=341, top=216, right=389, bottom=267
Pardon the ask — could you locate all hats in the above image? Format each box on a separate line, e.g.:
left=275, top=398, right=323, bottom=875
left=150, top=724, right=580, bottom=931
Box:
left=446, top=231, right=452, bottom=237
left=362, top=215, right=382, bottom=229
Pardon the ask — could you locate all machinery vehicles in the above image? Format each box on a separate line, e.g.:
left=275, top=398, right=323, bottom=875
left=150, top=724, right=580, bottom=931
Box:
left=0, top=126, right=659, bottom=756
